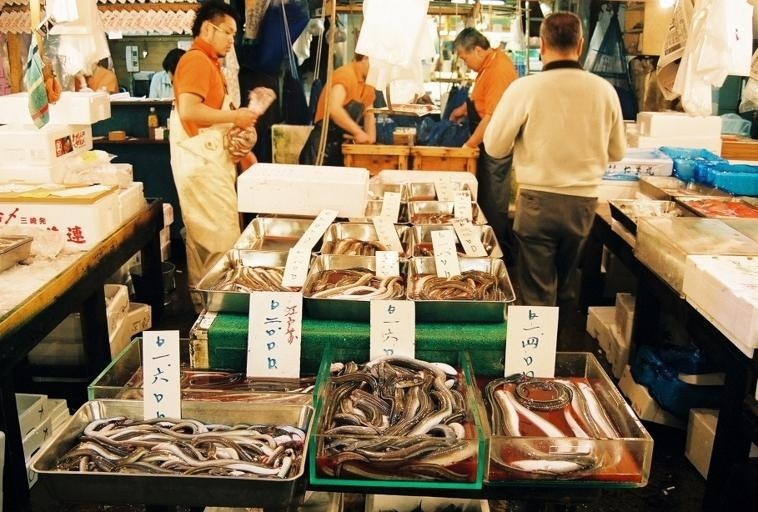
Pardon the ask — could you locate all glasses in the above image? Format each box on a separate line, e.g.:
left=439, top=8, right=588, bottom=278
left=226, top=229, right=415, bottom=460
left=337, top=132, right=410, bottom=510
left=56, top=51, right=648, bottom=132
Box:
left=207, top=20, right=237, bottom=38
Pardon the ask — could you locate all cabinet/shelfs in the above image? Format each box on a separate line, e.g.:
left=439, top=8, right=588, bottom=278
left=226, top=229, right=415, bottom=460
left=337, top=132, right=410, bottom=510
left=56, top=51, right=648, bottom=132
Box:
left=87, top=98, right=185, bottom=239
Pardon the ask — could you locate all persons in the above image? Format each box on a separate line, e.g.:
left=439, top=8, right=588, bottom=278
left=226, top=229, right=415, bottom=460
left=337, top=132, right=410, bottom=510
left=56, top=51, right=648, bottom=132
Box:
left=149, top=48, right=185, bottom=98
left=299, top=55, right=376, bottom=166
left=86, top=62, right=118, bottom=94
left=170, top=0, right=257, bottom=314
left=450, top=28, right=520, bottom=268
left=482, top=13, right=626, bottom=340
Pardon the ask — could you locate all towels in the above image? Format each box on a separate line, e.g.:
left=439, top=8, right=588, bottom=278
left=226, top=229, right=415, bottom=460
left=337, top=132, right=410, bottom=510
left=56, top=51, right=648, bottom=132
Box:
left=22, top=50, right=50, bottom=129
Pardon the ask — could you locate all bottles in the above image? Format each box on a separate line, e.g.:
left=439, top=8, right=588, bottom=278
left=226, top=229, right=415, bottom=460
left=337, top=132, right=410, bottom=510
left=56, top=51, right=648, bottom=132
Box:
left=147, top=107, right=158, bottom=140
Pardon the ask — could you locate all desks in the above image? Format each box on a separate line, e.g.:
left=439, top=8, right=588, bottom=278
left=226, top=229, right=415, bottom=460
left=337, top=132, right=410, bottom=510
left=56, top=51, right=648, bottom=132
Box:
left=191, top=215, right=515, bottom=377
left=594, top=118, right=758, bottom=511
left=0, top=196, right=164, bottom=510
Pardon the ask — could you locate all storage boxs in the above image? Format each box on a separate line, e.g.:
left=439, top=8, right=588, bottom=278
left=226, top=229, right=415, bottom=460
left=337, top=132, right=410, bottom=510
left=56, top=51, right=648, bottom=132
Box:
left=659, top=147, right=729, bottom=181
left=636, top=215, right=758, bottom=298
left=236, top=162, right=370, bottom=219
left=370, top=169, right=478, bottom=202
left=699, top=164, right=756, bottom=196
left=684, top=256, right=758, bottom=350
left=600, top=148, right=674, bottom=180
left=1, top=92, right=111, bottom=125
left=307, top=345, right=485, bottom=490
left=0, top=127, right=132, bottom=184
left=469, top=351, right=654, bottom=489
left=720, top=219, right=758, bottom=244
left=341, top=133, right=411, bottom=176
left=412, top=133, right=480, bottom=176
left=0, top=183, right=141, bottom=250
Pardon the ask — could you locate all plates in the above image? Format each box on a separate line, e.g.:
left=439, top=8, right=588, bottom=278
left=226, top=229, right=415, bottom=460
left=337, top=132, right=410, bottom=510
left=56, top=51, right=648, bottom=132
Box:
left=673, top=196, right=758, bottom=218
left=406, top=257, right=516, bottom=322
left=0, top=235, right=34, bottom=273
left=409, top=201, right=488, bottom=225
left=349, top=200, right=412, bottom=224
left=410, top=182, right=476, bottom=202
left=412, top=224, right=504, bottom=258
left=638, top=175, right=734, bottom=201
left=195, top=248, right=317, bottom=313
left=608, top=200, right=699, bottom=237
left=322, top=221, right=413, bottom=258
left=368, top=184, right=410, bottom=202
left=301, top=255, right=406, bottom=322
left=29, top=398, right=317, bottom=508
left=233, top=217, right=326, bottom=253
